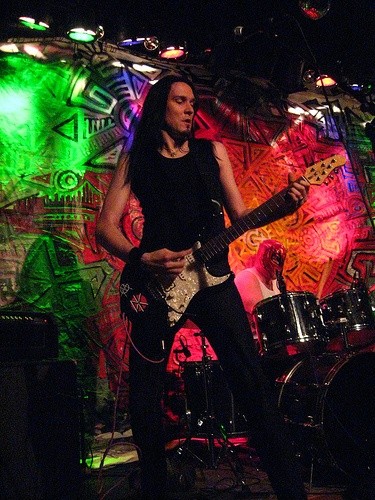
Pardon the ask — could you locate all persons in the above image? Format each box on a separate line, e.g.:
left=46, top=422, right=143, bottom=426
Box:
left=96, top=74, right=310, bottom=500
left=234, top=240, right=285, bottom=338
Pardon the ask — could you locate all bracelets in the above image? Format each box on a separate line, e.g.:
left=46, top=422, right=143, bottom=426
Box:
left=129, top=246, right=147, bottom=265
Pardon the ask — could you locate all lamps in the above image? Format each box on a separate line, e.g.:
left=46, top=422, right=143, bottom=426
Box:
left=144, top=31, right=162, bottom=51
left=134, top=19, right=146, bottom=43
left=14, top=2, right=51, bottom=33
left=159, top=35, right=189, bottom=63
left=65, top=10, right=101, bottom=44
left=118, top=16, right=133, bottom=46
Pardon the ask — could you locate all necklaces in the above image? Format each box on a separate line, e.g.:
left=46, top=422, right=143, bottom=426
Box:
left=165, top=148, right=183, bottom=157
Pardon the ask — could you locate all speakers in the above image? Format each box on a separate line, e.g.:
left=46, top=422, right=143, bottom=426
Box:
left=1, top=361, right=97, bottom=500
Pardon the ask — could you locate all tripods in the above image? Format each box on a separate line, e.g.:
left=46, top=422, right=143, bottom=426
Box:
left=163, top=333, right=251, bottom=493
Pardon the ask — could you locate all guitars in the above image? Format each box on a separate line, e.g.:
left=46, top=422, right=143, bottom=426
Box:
left=119, top=155, right=348, bottom=346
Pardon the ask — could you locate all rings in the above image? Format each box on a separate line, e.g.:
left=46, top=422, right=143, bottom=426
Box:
left=300, top=196, right=304, bottom=202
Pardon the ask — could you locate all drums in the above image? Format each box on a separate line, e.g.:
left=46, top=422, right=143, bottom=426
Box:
left=181, top=360, right=249, bottom=436
left=277, top=351, right=375, bottom=488
left=253, top=292, right=329, bottom=363
left=317, top=287, right=375, bottom=340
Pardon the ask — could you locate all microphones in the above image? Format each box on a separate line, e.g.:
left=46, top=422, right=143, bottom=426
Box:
left=180, top=339, right=191, bottom=357
